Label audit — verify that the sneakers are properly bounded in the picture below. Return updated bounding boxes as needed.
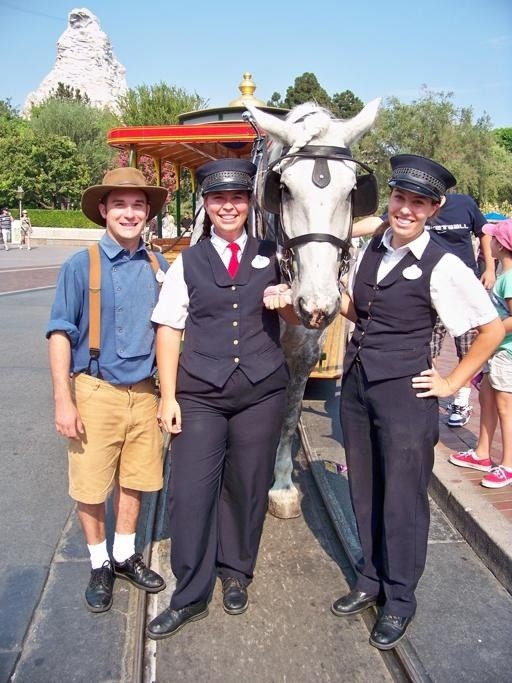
[449,448,492,472]
[447,400,473,428]
[481,462,512,488]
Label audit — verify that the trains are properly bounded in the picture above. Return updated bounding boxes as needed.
[101,66,360,383]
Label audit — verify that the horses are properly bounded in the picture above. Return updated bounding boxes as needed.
[188,95,384,520]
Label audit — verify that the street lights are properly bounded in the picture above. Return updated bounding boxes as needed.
[15,185,26,220]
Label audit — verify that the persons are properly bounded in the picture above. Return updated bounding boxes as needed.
[145,157,305,643]
[45,167,171,617]
[146,207,194,241]
[19,209,35,250]
[351,195,512,488]
[0,209,15,251]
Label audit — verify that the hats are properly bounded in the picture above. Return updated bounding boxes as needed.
[482,218,512,252]
[196,158,257,195]
[79,167,168,226]
[387,153,458,205]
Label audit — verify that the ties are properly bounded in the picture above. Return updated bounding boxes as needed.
[225,238,243,276]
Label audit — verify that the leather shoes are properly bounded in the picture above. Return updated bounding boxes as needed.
[222,574,250,614]
[330,586,374,614]
[368,604,416,650]
[110,552,166,593]
[146,600,209,641]
[83,565,115,613]
